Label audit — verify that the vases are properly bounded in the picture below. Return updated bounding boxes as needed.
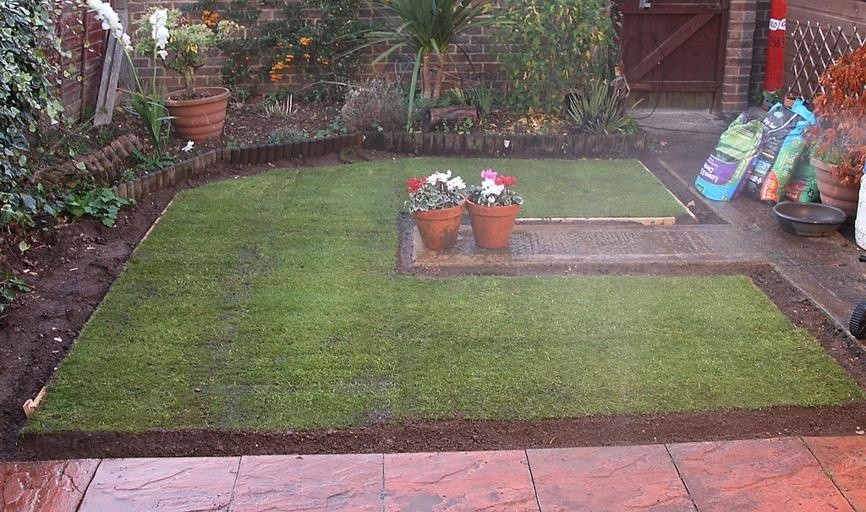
[164,86,232,142]
[809,154,863,225]
[410,198,523,250]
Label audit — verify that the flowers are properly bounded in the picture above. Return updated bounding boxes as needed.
[803,38,866,184]
[405,165,518,214]
[131,4,239,95]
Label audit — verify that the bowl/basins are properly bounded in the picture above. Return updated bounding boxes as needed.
[771,200,847,238]
